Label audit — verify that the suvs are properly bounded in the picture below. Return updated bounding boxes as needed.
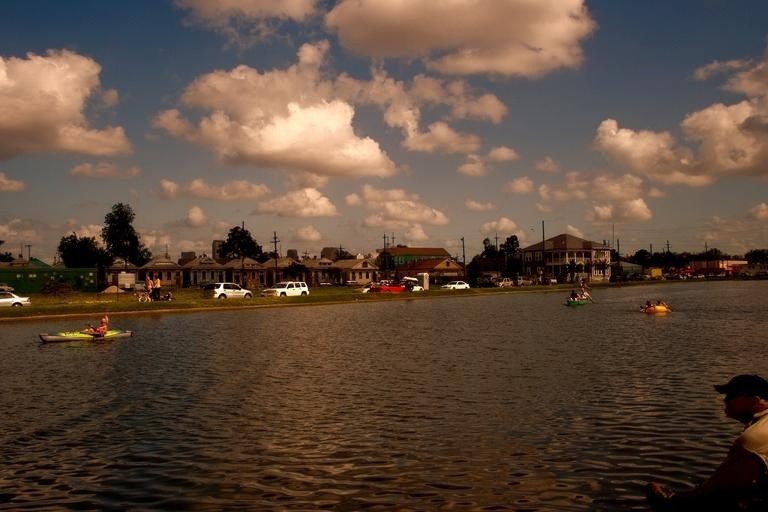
[261,281,310,298]
[203,281,254,300]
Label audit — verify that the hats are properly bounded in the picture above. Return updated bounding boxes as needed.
[711,373,768,399]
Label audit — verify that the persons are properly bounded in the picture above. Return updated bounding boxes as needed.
[80,314,108,337]
[407,280,414,293]
[571,280,592,301]
[145,274,160,301]
[639,296,672,313]
[646,374,768,512]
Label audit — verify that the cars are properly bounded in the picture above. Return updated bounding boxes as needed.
[0,292,33,308]
[441,280,470,291]
[319,282,334,287]
[359,276,425,293]
[663,272,706,280]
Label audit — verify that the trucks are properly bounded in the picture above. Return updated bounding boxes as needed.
[642,268,662,281]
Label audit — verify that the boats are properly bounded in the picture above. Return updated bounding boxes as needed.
[39,328,134,344]
[644,304,672,314]
[566,297,591,306]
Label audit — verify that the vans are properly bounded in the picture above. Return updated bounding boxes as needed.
[344,281,359,285]
[495,277,513,288]
[517,276,534,286]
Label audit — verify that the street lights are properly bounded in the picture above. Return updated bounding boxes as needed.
[461,236,467,283]
